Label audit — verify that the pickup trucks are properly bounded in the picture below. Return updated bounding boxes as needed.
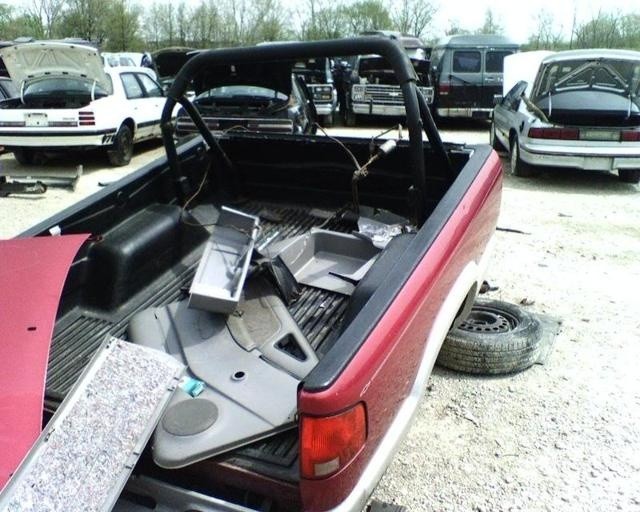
[9,36,505,512]
